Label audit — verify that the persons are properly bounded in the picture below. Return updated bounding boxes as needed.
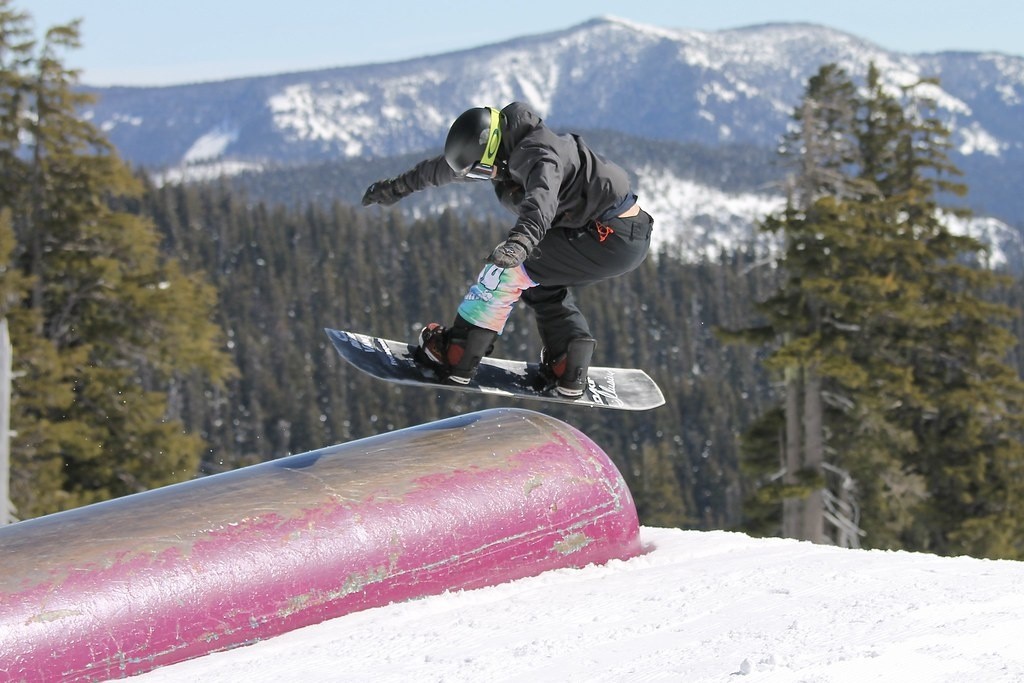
[362,100,655,399]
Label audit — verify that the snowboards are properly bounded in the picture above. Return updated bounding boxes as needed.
[323,325,666,412]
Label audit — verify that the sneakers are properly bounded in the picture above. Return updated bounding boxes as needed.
[537,354,585,398]
[418,322,475,385]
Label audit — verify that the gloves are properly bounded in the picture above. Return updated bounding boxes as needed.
[361,182,381,207]
[485,234,533,268]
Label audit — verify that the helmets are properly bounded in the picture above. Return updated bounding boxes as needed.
[444,108,506,178]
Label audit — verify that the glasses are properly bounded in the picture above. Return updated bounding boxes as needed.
[465,162,493,181]
